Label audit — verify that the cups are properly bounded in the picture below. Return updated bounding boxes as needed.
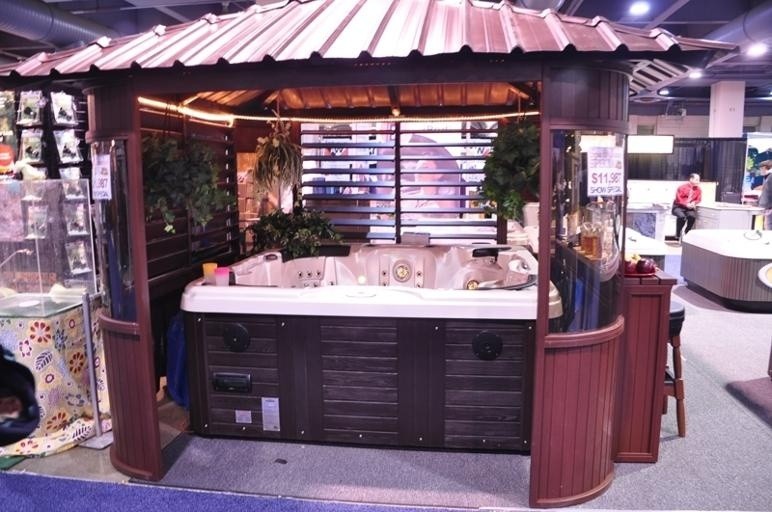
[214,266,230,286]
[202,262,217,284]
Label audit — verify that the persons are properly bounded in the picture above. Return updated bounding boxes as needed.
[758,159,771,231]
[671,172,702,243]
[393,152,450,220]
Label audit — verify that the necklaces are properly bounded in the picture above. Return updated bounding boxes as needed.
[414,194,435,208]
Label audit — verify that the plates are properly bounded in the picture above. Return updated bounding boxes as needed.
[624,272,656,277]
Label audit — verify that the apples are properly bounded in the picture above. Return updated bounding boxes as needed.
[637,260,653,273]
[626,261,638,273]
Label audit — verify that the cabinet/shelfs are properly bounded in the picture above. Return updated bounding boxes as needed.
[695,207,719,230]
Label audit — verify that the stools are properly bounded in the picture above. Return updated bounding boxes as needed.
[665,300,686,436]
